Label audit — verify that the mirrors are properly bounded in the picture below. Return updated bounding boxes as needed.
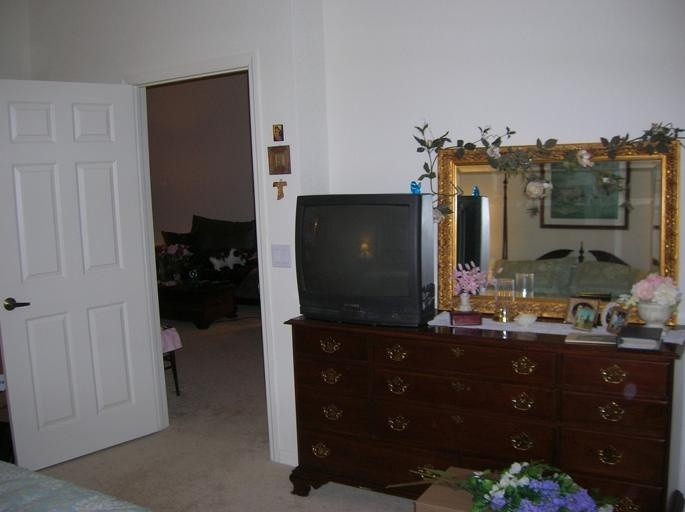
[436,137,681,324]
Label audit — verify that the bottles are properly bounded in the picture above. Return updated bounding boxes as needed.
[471,186,481,196]
[410,181,421,194]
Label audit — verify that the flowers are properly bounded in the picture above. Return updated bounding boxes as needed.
[154,243,194,273]
[383,458,621,512]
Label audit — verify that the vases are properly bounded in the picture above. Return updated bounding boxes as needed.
[158,262,176,283]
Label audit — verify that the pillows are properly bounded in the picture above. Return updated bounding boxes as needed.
[487,259,640,296]
[161,225,253,281]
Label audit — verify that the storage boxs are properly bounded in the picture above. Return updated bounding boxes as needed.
[413,466,501,512]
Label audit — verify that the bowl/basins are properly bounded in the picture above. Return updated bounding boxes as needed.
[514,314,537,327]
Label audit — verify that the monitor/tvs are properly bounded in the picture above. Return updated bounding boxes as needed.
[458,195,488,295]
[294,192,436,328]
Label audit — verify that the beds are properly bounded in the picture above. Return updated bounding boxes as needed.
[485,251,647,303]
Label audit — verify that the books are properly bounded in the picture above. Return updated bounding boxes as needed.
[616,325,663,351]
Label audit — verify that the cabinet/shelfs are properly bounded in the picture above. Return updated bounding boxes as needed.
[282,299,685,512]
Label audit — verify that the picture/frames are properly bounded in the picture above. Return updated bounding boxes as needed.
[538,160,631,231]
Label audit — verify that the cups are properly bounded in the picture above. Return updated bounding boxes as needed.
[516,272,535,299]
[493,279,515,323]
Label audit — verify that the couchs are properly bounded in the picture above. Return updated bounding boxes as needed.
[157,214,260,304]
